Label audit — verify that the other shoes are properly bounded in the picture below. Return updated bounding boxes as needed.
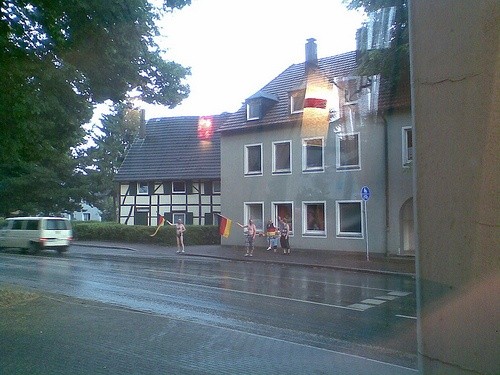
[249,254,252,257]
[244,254,249,257]
[287,249,290,253]
[283,249,286,254]
[181,250,185,253]
[266,247,272,251]
[176,251,181,253]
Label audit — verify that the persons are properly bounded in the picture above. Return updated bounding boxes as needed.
[279,217,291,255]
[166,218,186,253]
[266,219,278,251]
[236,218,256,257]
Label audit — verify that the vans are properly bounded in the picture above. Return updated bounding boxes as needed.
[0,216,73,254]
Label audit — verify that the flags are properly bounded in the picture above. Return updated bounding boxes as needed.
[218,217,232,238]
[150,216,164,236]
[266,227,276,236]
